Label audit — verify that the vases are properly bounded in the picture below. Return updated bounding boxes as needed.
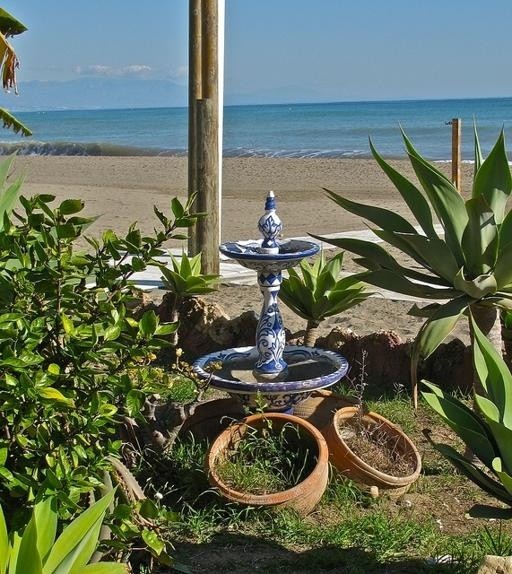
[205,412,330,521]
[293,389,334,419]
[319,406,423,503]
